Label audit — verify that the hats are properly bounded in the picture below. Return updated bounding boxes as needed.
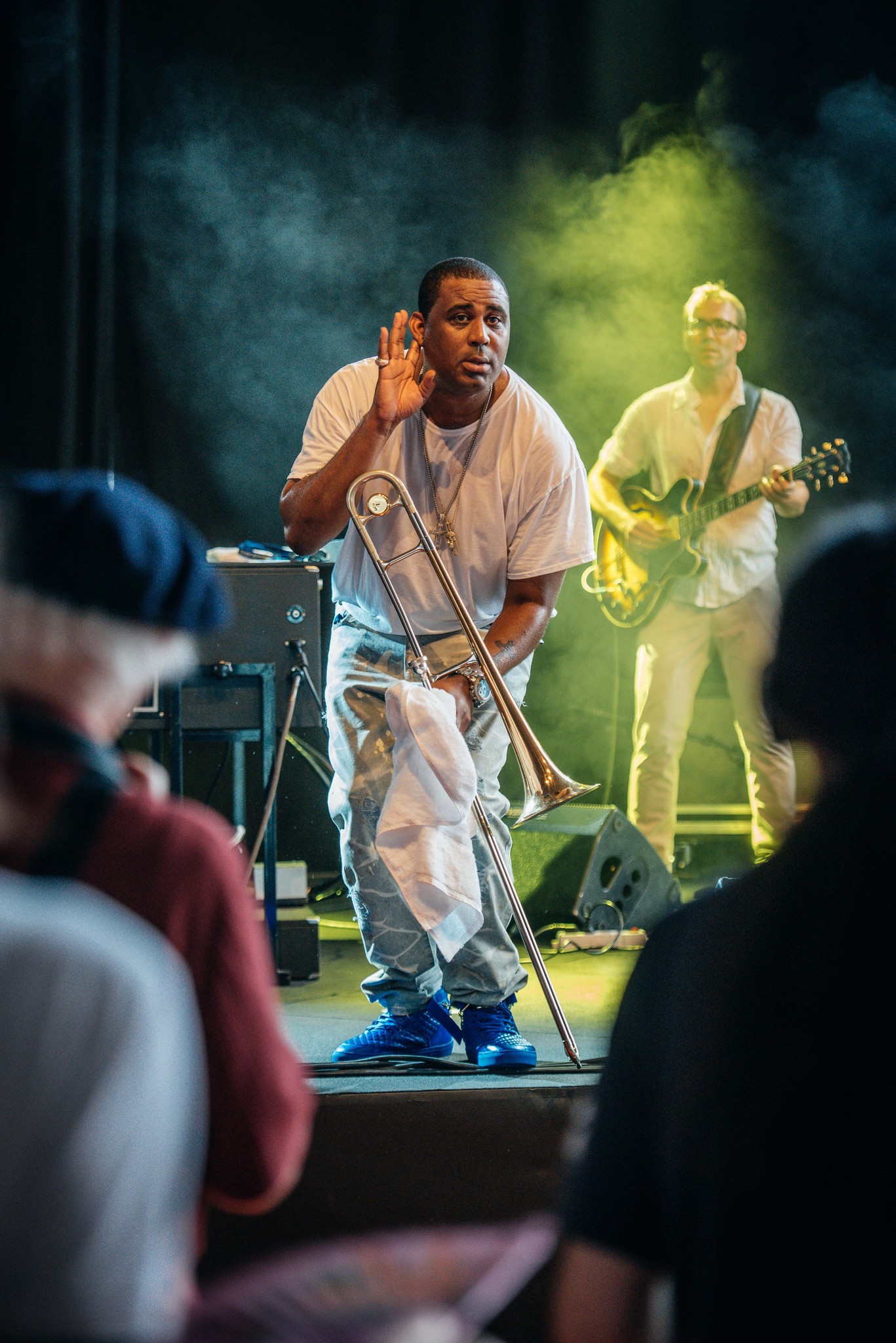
[763,504,896,746]
[0,469,231,638]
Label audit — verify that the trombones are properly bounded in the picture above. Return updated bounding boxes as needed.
[346,469,606,1071]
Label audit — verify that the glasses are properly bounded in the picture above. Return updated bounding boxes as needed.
[684,316,741,335]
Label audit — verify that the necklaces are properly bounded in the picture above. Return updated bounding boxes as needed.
[415,382,493,556]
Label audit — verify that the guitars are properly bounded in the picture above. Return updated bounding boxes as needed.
[590,437,853,628]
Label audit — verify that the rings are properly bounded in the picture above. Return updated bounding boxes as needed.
[374,358,389,366]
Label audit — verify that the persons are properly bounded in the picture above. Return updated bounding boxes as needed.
[279,256,597,1069]
[0,871,208,1343]
[553,517,896,1343]
[0,465,317,1343]
[586,282,809,873]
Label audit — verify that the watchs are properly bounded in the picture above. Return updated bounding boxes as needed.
[446,665,492,708]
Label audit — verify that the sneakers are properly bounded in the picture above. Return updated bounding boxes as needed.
[456,1003,536,1066]
[332,990,462,1064]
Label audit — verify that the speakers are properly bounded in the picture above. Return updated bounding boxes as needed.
[500,800,685,946]
[128,548,321,729]
[193,1074,602,1297]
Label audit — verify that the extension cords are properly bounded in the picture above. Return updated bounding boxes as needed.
[555,927,648,956]
